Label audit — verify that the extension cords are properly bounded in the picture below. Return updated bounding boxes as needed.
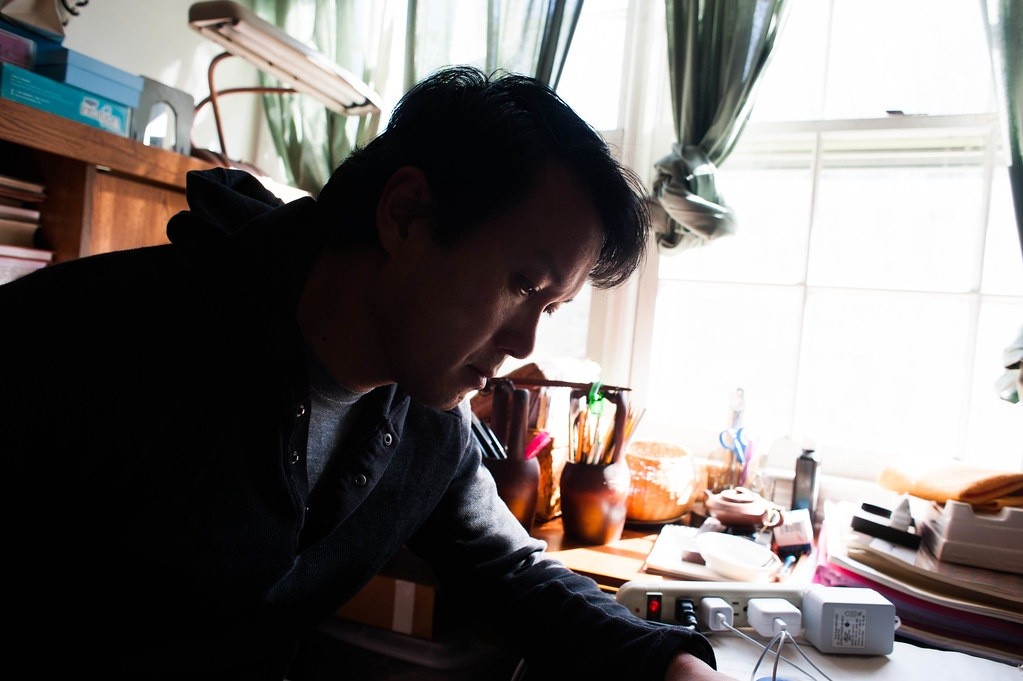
[617,581,824,632]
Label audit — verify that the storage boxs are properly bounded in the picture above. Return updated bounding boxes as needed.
[0,61,128,137]
[45,47,144,109]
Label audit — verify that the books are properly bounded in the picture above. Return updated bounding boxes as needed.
[641,478,1023,672]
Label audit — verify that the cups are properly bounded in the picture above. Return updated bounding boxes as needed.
[559,456,629,549]
[477,453,540,538]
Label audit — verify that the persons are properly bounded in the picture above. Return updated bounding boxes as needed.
[0,62,752,681]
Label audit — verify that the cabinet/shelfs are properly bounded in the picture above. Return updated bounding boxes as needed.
[0,98,229,286]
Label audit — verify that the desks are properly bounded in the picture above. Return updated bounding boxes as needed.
[545,532,1023,681]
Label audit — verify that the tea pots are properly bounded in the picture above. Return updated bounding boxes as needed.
[701,485,784,529]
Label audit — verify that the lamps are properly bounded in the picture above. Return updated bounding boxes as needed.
[188,0,383,182]
